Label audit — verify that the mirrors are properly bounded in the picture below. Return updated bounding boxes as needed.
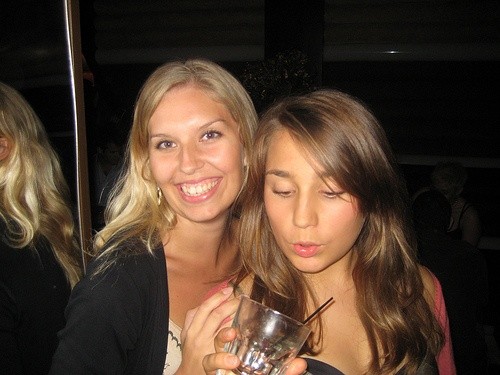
[0,0,93,375]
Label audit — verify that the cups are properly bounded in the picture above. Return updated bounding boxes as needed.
[216,295,311,375]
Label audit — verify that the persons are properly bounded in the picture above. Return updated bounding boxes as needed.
[53,59,259,375]
[206,89,454,374]
[0,82,86,375]
